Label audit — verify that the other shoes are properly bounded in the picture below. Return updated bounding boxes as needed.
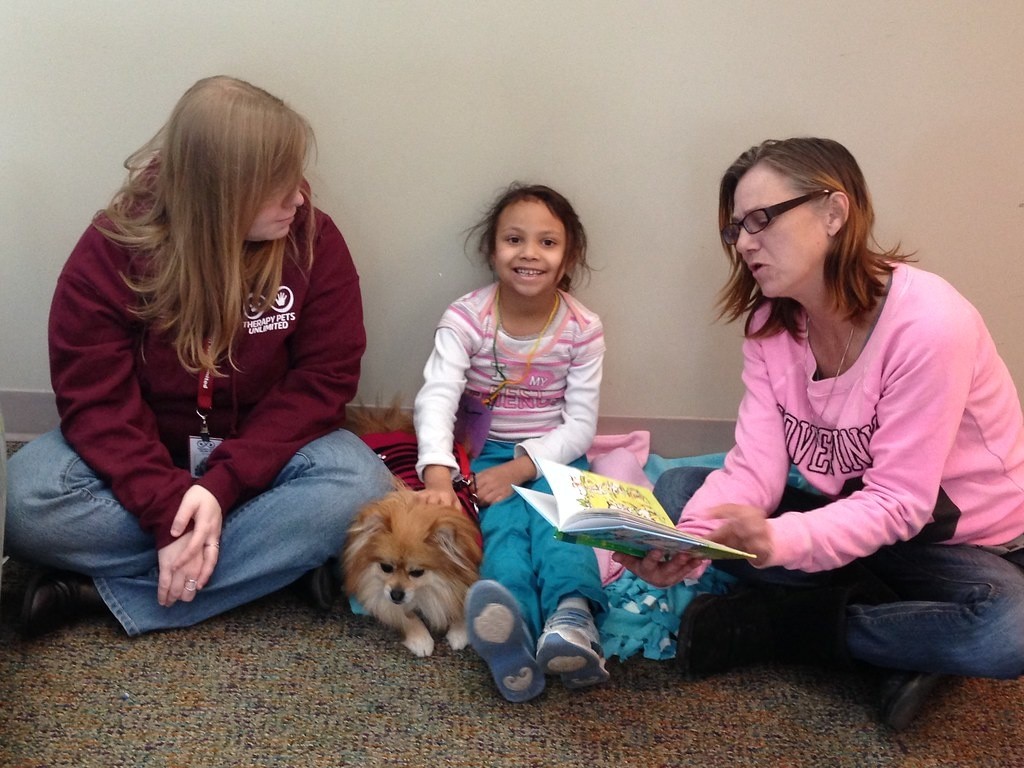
[676,582,836,679]
[812,599,939,731]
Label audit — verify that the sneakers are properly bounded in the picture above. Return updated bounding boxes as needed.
[464,580,545,703]
[536,606,608,691]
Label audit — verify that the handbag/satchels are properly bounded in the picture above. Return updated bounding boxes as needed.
[361,431,483,563]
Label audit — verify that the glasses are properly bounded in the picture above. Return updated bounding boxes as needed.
[719,189,836,245]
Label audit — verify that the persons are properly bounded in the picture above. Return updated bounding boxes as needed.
[415,179,610,703]
[1,75,398,657]
[610,136,1023,729]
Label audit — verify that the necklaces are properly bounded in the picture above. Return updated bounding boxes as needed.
[802,295,856,445]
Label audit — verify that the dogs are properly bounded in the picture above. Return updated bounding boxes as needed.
[342,388,483,658]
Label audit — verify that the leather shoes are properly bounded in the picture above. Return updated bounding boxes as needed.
[284,553,340,613]
[20,563,111,643]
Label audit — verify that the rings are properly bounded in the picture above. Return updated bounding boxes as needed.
[183,579,197,590]
[205,542,220,549]
